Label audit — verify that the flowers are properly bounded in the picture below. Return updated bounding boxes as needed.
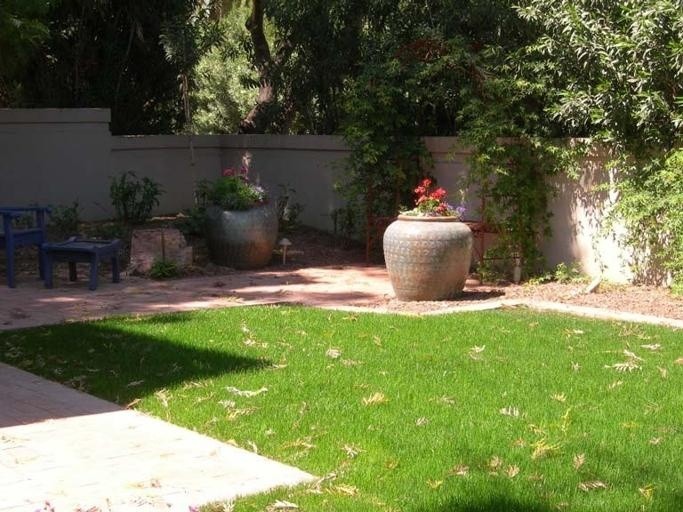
[197,147,272,211]
[397,177,466,219]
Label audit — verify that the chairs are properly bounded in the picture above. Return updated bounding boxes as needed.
[0,205,52,288]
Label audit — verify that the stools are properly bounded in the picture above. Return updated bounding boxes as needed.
[42,235,123,292]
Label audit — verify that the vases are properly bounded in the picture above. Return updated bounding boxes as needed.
[219,206,278,266]
[381,214,475,302]
